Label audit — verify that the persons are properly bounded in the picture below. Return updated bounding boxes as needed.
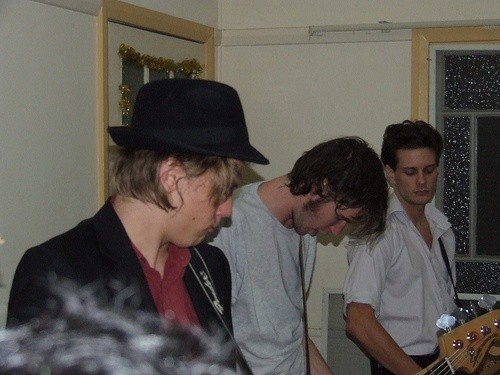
[4,78,254,375]
[204,136,390,375]
[0,278,237,375]
[343,119,457,375]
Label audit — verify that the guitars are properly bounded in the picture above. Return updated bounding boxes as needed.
[415,293,500,375]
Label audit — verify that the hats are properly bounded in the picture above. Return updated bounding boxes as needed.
[107,77,270,164]
